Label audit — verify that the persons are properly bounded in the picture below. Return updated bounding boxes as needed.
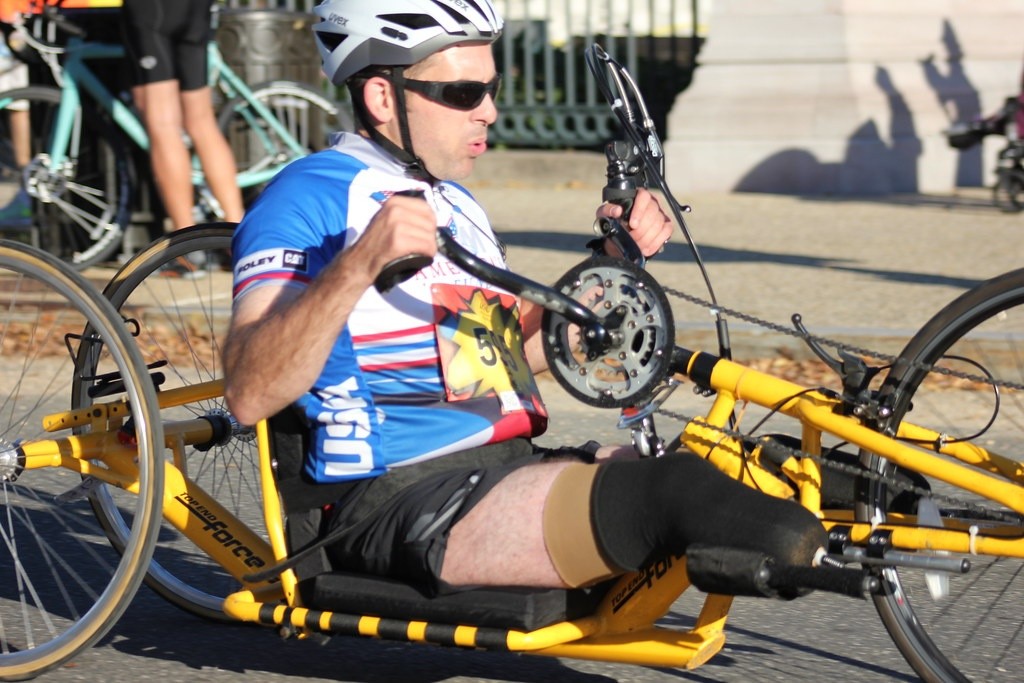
[0,0,246,280]
[221,0,934,600]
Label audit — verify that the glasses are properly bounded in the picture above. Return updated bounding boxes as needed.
[358,68,503,111]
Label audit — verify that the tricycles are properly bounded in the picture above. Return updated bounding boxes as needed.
[0,39,1024,683]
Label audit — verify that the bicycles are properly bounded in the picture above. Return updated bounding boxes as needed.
[2,9,358,278]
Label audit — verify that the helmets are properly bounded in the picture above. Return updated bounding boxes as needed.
[309,0,504,87]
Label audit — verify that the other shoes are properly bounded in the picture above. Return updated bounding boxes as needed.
[116,247,206,280]
